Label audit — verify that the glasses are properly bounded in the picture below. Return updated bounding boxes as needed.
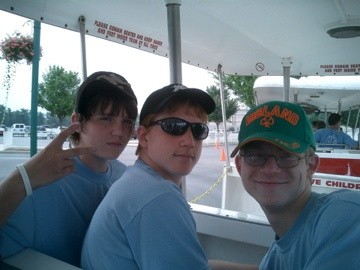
[240,150,309,170]
[150,116,209,142]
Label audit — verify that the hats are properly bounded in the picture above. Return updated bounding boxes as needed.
[138,83,216,119]
[229,100,315,153]
[74,71,135,101]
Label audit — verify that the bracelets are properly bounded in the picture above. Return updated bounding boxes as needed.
[16,163,32,196]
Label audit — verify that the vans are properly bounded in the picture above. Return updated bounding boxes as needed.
[29,126,47,140]
[11,123,25,137]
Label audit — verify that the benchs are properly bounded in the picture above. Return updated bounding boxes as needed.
[315,143,360,177]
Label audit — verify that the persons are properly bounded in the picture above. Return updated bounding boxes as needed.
[310,113,360,149]
[80,82,259,270]
[229,100,360,270]
[0,70,139,270]
